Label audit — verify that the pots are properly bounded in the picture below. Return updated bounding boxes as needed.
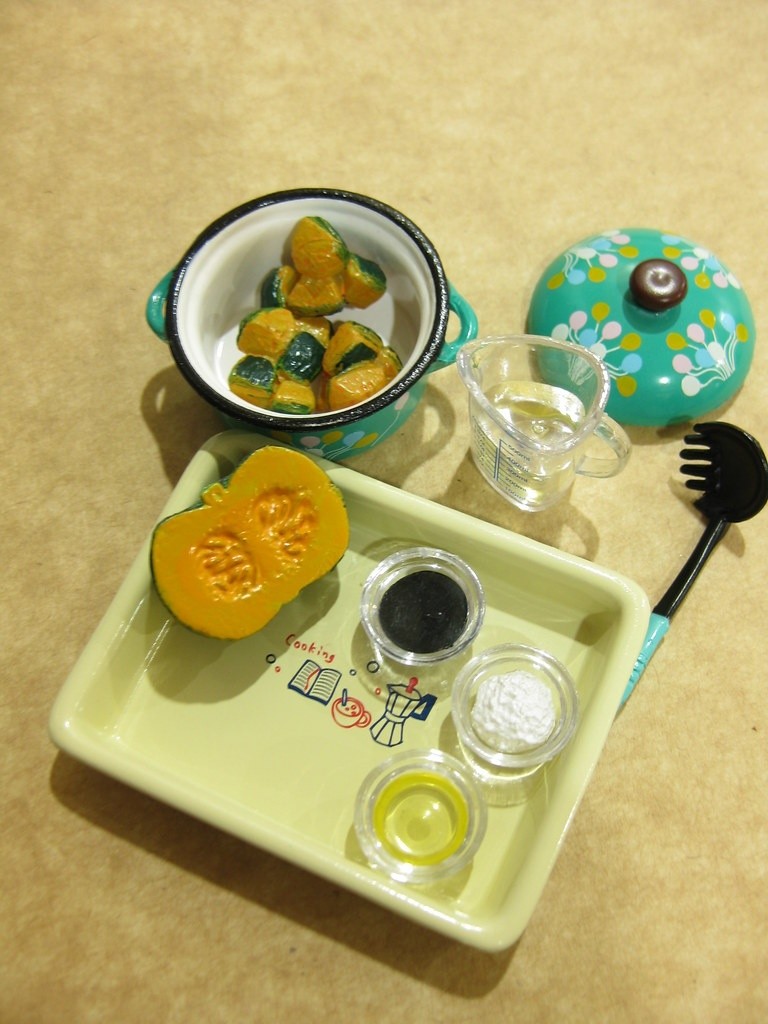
[144,188,479,462]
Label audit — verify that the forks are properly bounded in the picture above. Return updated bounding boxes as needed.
[621,421,768,704]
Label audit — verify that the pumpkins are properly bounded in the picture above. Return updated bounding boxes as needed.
[227,218,408,417]
[148,444,350,641]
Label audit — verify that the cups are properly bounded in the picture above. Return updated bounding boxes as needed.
[456,335,632,513]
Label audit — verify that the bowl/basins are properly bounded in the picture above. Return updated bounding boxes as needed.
[359,546,485,665]
[354,749,487,886]
[449,642,580,768]
